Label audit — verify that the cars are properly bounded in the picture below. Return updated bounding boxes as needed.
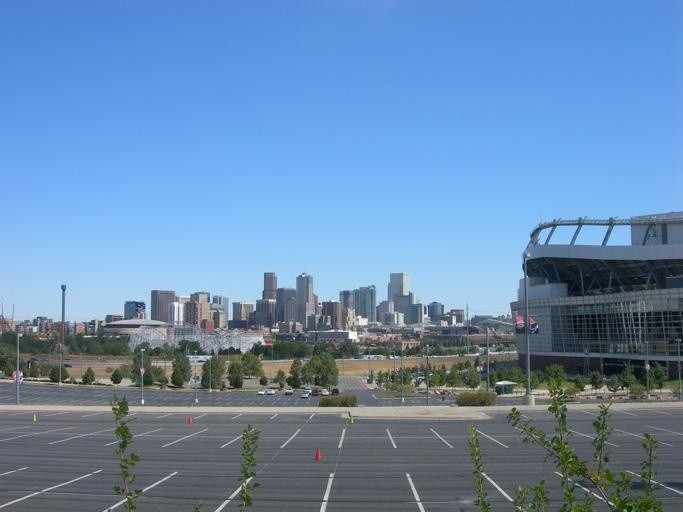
[257,385,340,398]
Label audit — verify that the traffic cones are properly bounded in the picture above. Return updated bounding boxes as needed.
[188,416,191,424]
[316,448,320,461]
